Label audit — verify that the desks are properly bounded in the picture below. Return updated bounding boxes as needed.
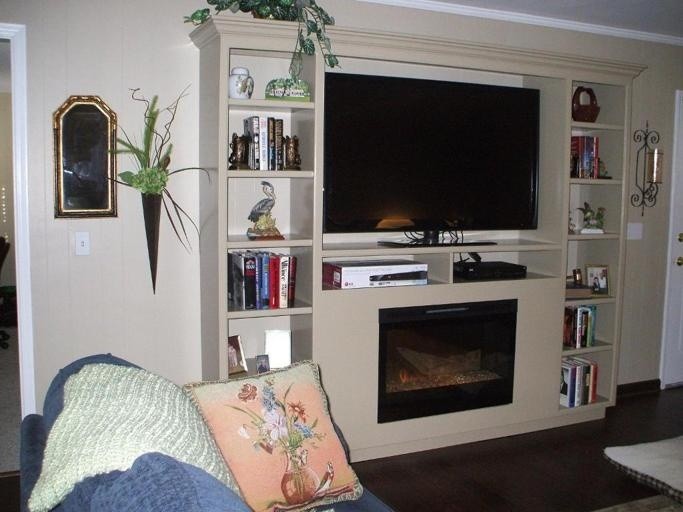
[602,438,683,500]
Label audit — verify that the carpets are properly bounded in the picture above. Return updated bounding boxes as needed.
[592,494,683,512]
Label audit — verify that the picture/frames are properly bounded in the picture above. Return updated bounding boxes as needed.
[256,354,270,374]
[584,263,611,298]
[229,335,249,375]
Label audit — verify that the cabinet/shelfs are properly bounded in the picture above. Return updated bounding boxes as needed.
[200,47,648,462]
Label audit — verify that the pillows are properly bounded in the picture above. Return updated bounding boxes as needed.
[182,360,365,511]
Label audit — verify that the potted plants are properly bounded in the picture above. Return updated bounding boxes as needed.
[183,0,342,99]
[108,88,211,295]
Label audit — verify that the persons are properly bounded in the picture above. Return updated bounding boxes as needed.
[599,272,607,288]
[592,276,600,294]
[560,369,568,396]
[257,359,268,373]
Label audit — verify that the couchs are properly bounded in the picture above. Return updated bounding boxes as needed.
[18,356,394,512]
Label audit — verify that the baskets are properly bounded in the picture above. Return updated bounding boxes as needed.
[572,87,601,122]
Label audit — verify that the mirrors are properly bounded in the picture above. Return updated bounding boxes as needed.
[51,93,118,217]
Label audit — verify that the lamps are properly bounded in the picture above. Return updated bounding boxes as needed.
[631,121,664,216]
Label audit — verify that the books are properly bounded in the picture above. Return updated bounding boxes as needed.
[570,135,599,180]
[558,304,598,408]
[227,250,298,311]
[243,116,284,172]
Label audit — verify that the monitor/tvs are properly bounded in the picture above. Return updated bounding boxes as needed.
[324,72,541,249]
